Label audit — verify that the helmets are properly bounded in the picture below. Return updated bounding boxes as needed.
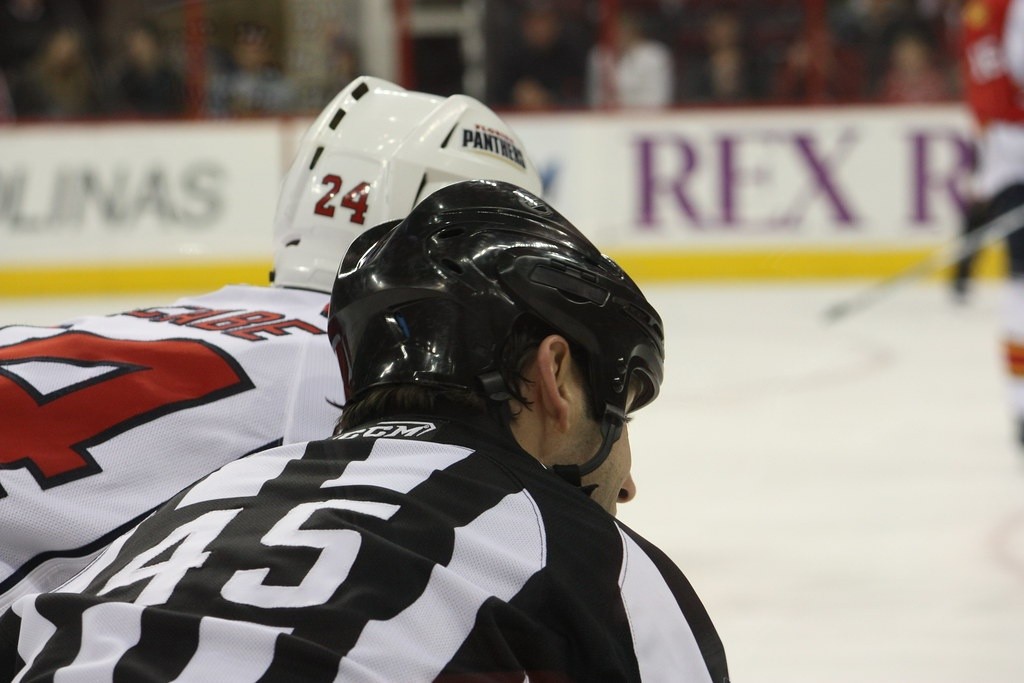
[327,179,665,424]
[271,76,543,297]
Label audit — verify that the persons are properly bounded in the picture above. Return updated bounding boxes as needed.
[0,74,542,613]
[0,21,358,120]
[0,179,730,683]
[945,0,1024,444]
[484,0,958,111]
